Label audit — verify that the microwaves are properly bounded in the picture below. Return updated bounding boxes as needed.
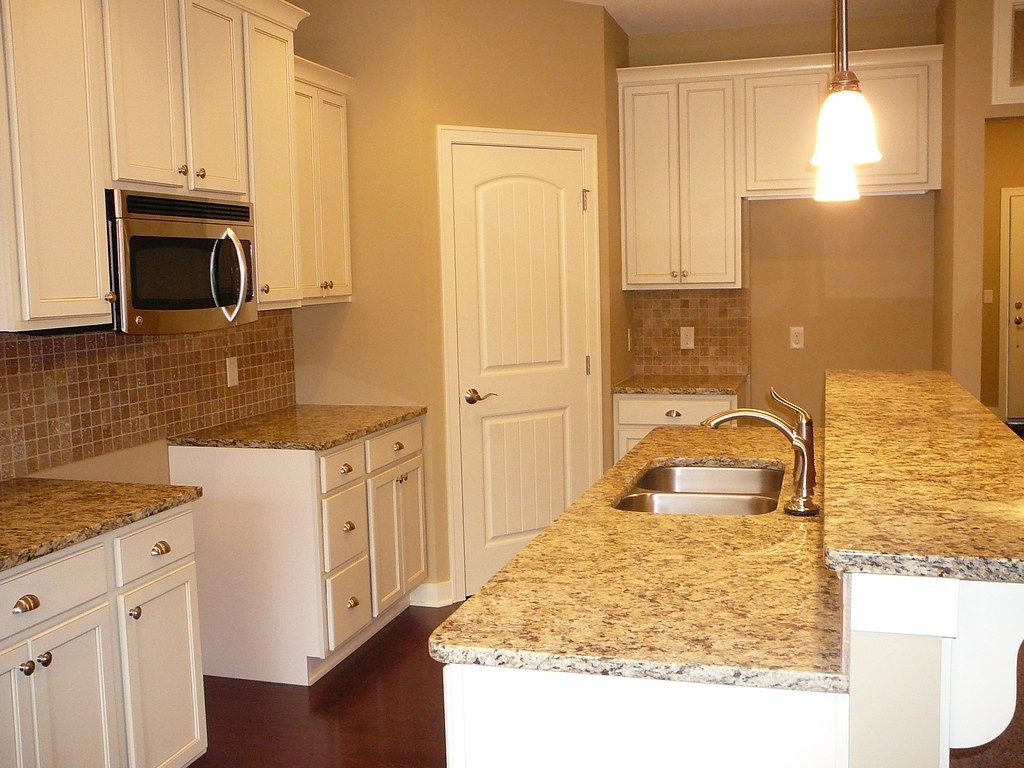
[111,188,259,336]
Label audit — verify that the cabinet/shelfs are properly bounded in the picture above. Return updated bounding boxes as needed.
[616,44,943,290]
[168,416,428,686]
[0,0,353,334]
[612,380,747,466]
[0,501,208,768]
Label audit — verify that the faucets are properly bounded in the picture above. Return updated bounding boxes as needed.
[699,384,823,518]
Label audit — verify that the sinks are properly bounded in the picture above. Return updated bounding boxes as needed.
[609,493,780,517]
[632,455,788,493]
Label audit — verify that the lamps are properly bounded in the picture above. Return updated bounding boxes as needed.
[813,0,859,202]
[810,0,884,166]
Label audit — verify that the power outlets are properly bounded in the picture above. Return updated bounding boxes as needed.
[790,326,804,349]
[680,327,694,349]
